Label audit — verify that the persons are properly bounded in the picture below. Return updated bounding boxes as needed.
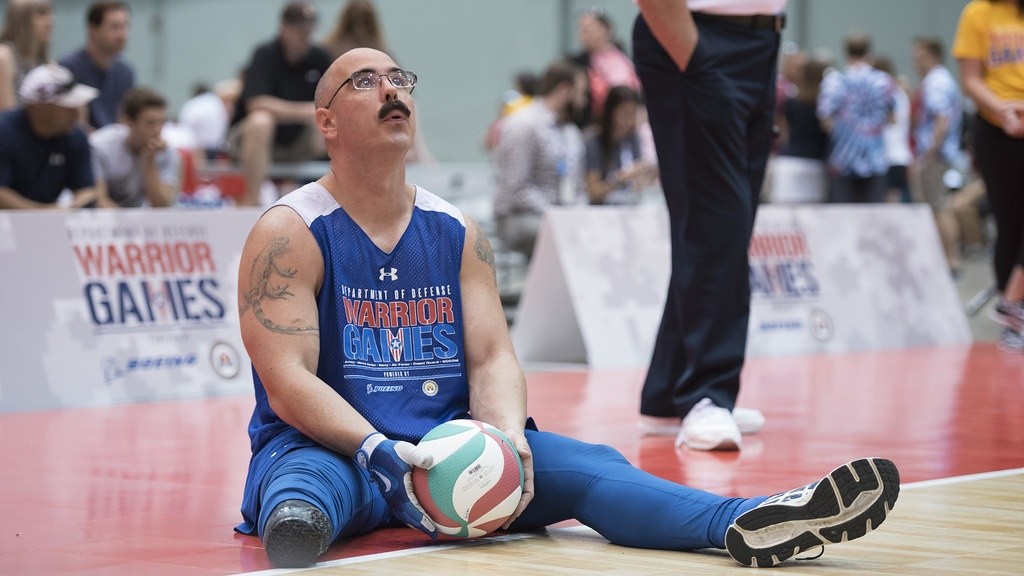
[0,0,990,277]
[949,0,1024,341]
[232,46,899,568]
[631,1,788,450]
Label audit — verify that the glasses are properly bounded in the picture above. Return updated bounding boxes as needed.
[324,70,417,109]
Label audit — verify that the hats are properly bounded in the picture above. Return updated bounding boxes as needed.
[283,3,316,22]
[21,63,98,108]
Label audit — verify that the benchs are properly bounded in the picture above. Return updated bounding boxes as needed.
[189,157,332,203]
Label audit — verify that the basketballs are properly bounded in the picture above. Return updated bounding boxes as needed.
[412,418,526,540]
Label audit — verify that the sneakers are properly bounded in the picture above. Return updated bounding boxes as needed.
[266,500,330,567]
[986,295,1024,336]
[638,405,765,434]
[676,399,740,451]
[726,458,901,567]
[997,326,1024,354]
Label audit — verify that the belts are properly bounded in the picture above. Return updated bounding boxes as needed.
[694,11,786,31]
